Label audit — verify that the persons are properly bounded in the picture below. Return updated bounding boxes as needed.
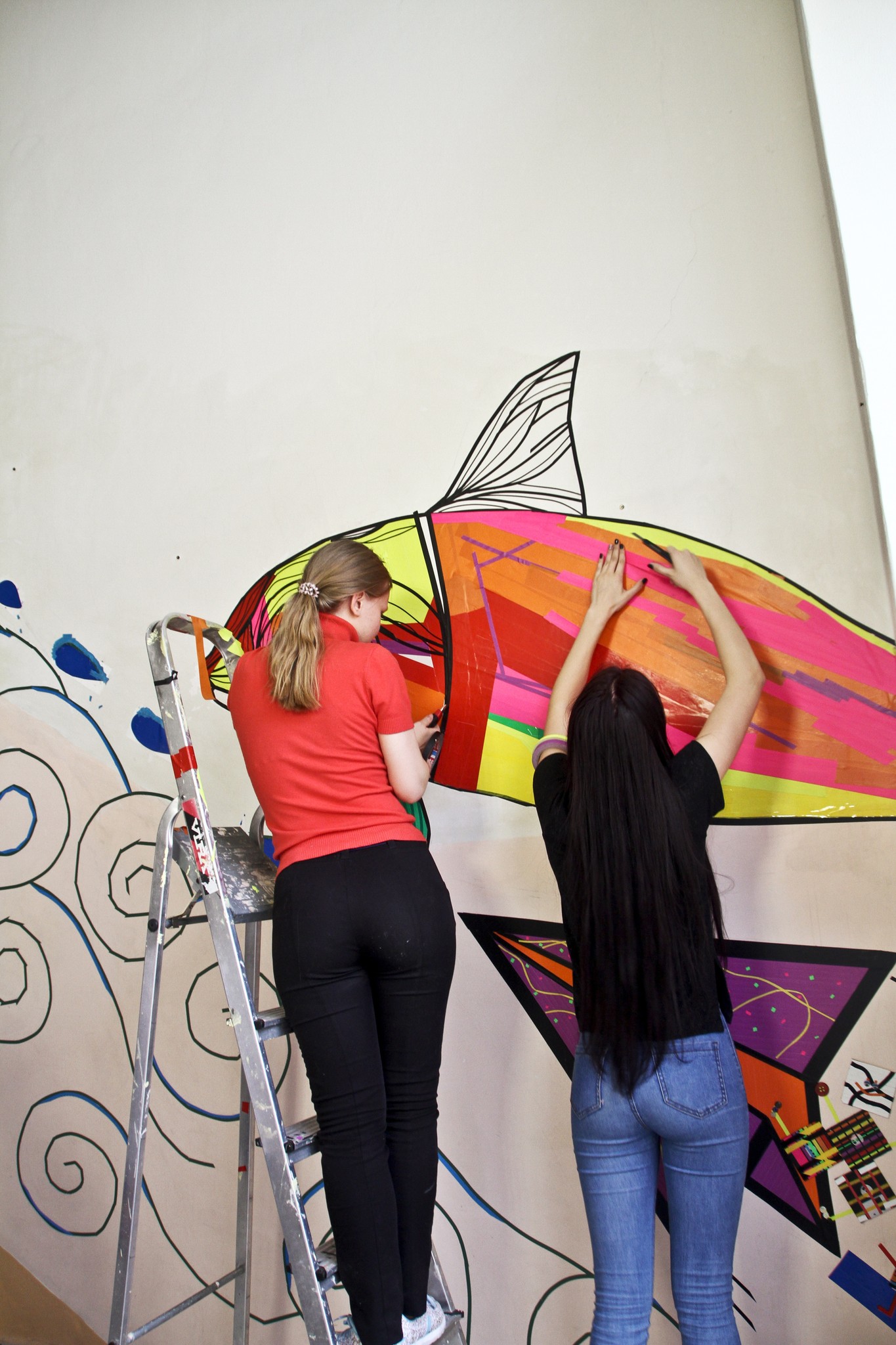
[228,542,449,1344]
[531,536,767,1345]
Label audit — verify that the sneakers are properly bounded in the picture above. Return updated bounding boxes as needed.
[400,1293,445,1345]
[335,1326,409,1345]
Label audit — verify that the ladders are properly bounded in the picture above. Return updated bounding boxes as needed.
[106,616,466,1345]
